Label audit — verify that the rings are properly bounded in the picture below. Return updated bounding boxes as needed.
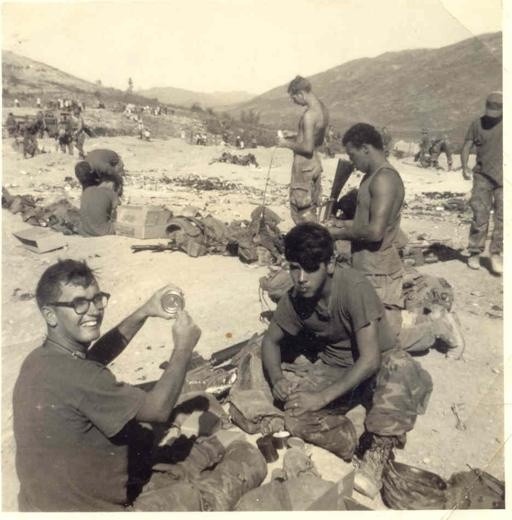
[293,403,299,409]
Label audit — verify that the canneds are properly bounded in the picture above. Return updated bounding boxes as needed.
[286,435,305,451]
[159,290,186,316]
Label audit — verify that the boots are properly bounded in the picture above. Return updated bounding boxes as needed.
[354,436,392,499]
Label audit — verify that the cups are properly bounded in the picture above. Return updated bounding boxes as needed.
[256,430,290,464]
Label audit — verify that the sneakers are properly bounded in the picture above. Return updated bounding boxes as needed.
[491,254,503,273]
[284,449,319,478]
[467,253,480,268]
[430,306,464,359]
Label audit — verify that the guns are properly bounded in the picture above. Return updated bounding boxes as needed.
[132,331,268,391]
[130,245,177,252]
[321,159,355,225]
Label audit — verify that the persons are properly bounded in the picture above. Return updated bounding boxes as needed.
[273,74,329,222]
[412,126,453,172]
[316,123,348,160]
[10,258,267,511]
[461,89,503,278]
[192,133,207,146]
[379,126,394,158]
[5,95,169,238]
[259,223,435,499]
[220,131,260,150]
[324,121,464,361]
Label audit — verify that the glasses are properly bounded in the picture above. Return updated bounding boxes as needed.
[48,292,110,314]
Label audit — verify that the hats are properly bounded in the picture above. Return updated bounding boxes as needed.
[485,93,502,118]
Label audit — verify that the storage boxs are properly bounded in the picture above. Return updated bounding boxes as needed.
[111,203,171,239]
